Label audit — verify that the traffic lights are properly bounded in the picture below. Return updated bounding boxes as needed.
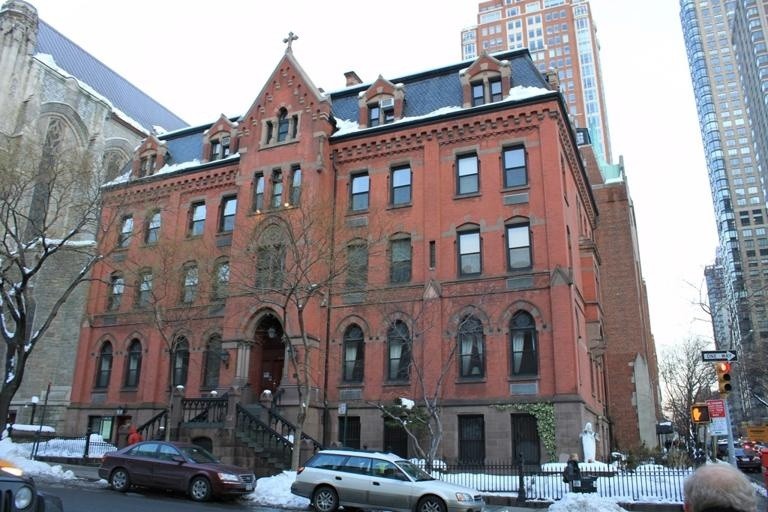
[690,404,710,424]
[715,361,732,398]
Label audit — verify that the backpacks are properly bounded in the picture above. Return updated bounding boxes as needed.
[563,467,571,483]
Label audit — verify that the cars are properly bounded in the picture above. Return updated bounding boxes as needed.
[98,441,257,502]
[718,439,768,473]
[290,449,486,512]
[0,467,63,512]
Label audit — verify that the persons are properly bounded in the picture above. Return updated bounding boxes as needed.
[682,464,758,512]
[128,424,144,445]
[567,453,583,494]
